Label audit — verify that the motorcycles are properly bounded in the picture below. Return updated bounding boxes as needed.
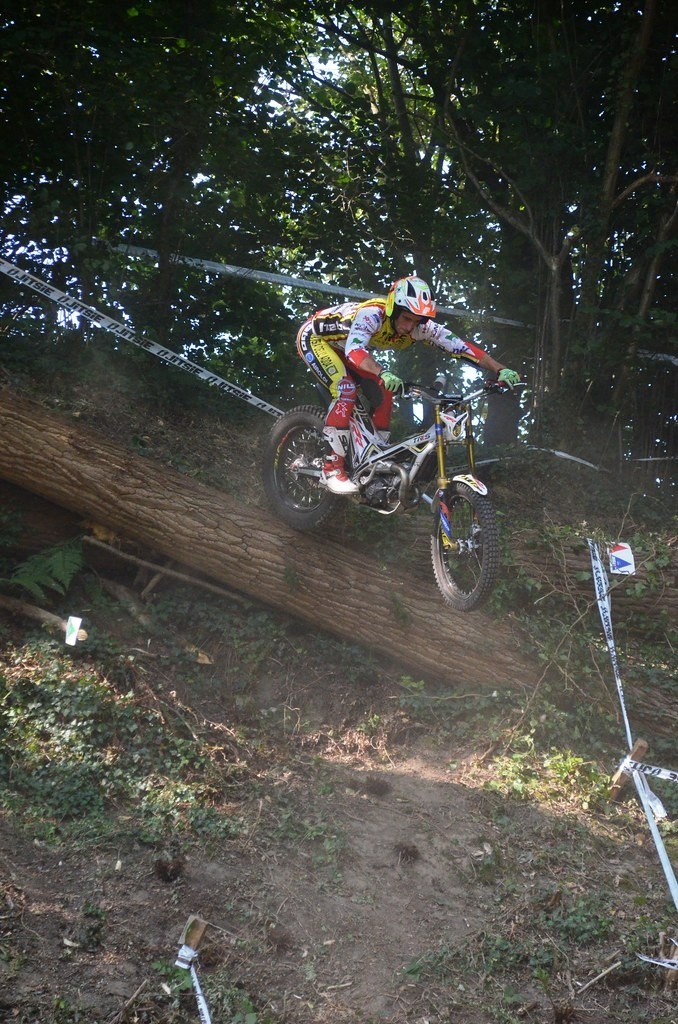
[262,367,530,613]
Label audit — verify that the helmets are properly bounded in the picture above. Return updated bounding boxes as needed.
[385,276,437,324]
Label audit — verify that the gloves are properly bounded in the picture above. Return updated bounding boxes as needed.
[378,368,405,399]
[496,367,521,391]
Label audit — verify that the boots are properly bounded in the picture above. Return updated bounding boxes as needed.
[378,430,391,444]
[318,426,360,495]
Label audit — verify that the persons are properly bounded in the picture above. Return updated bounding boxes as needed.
[296,277,521,494]
